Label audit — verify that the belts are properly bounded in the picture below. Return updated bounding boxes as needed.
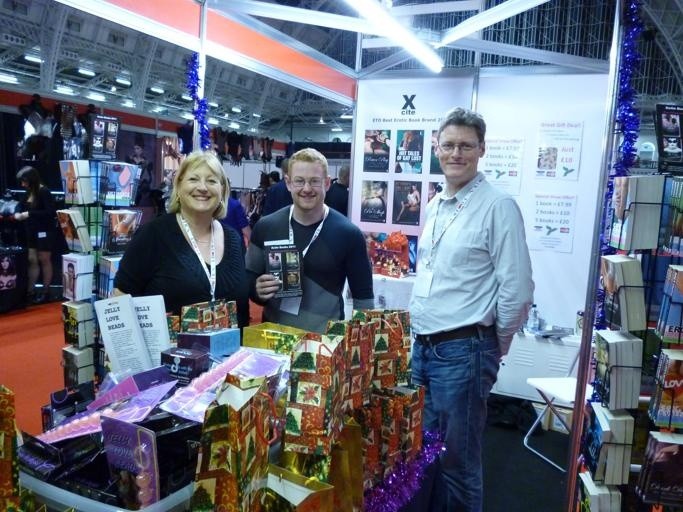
[416,324,496,345]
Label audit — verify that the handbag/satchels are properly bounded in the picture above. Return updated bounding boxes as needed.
[167,299,238,343]
[189,376,268,512]
[1,385,19,512]
[244,322,305,354]
[268,308,424,512]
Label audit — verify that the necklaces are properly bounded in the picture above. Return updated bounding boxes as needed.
[184,223,217,244]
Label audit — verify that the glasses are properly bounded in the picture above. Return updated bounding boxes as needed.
[292,175,326,187]
[437,141,480,151]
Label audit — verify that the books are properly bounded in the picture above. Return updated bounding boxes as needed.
[135,295,170,368]
[655,102,683,176]
[91,293,153,383]
[263,240,302,299]
[86,112,122,162]
[575,171,683,511]
[57,158,143,388]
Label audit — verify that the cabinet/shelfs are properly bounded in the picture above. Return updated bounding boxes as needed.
[567,110,683,512]
[61,148,140,398]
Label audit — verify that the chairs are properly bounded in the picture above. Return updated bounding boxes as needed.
[525,337,585,475]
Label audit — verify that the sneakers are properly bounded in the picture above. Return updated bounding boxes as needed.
[27,293,51,301]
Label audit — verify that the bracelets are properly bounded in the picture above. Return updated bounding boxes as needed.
[383,138,389,143]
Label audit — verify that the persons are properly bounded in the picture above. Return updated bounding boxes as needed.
[395,184,420,222]
[18,93,54,160]
[409,106,534,508]
[128,134,148,165]
[107,141,115,150]
[363,130,389,154]
[0,257,17,289]
[242,146,376,337]
[663,137,682,154]
[218,153,351,243]
[21,165,56,267]
[93,137,103,149]
[362,181,387,208]
[111,151,252,336]
